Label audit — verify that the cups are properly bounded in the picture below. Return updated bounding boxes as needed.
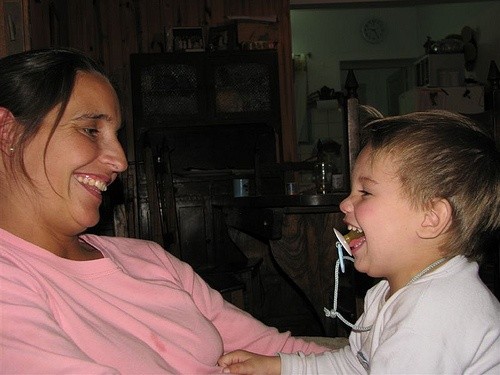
[283,171,300,207]
[332,175,343,190]
[313,162,332,194]
[232,179,250,197]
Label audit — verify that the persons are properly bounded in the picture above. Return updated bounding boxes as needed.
[217,109,500,375]
[0,48,331,375]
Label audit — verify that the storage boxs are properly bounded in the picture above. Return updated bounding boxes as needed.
[229,19,279,48]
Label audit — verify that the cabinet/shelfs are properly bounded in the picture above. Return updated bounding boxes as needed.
[124,49,283,318]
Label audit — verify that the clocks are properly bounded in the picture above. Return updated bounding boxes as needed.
[361,16,386,43]
